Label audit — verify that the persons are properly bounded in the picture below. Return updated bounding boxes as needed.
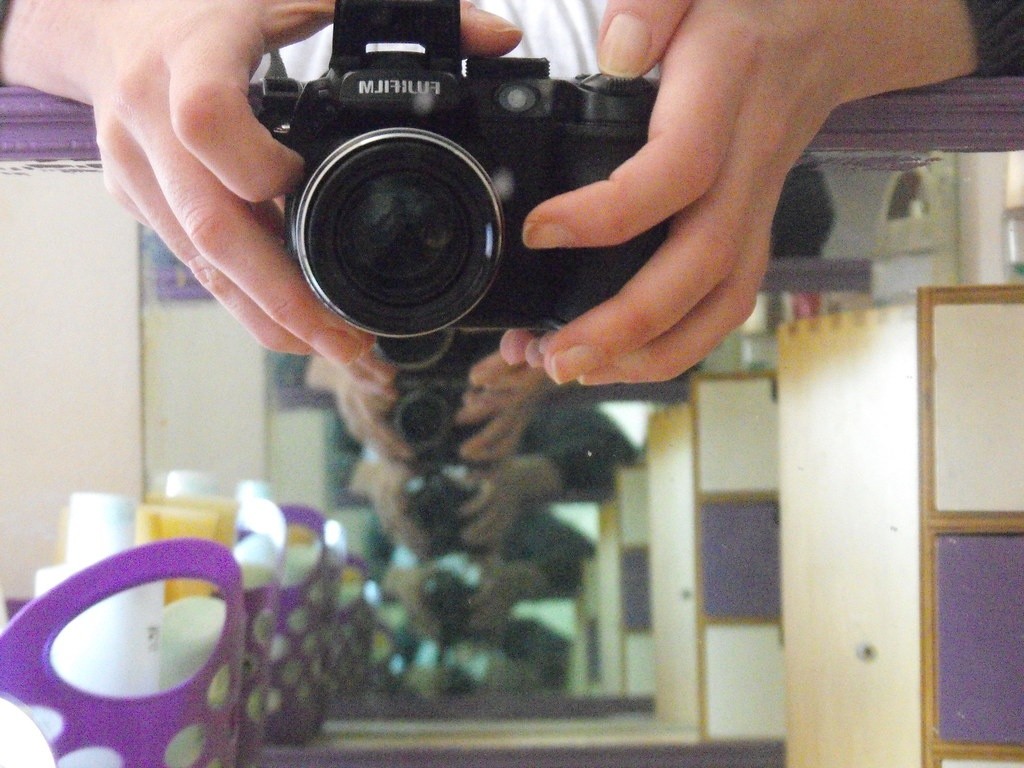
[265,351,639,697]
[1,0,1024,391]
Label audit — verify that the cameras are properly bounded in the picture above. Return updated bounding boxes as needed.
[252,0,669,341]
[370,337,489,710]
